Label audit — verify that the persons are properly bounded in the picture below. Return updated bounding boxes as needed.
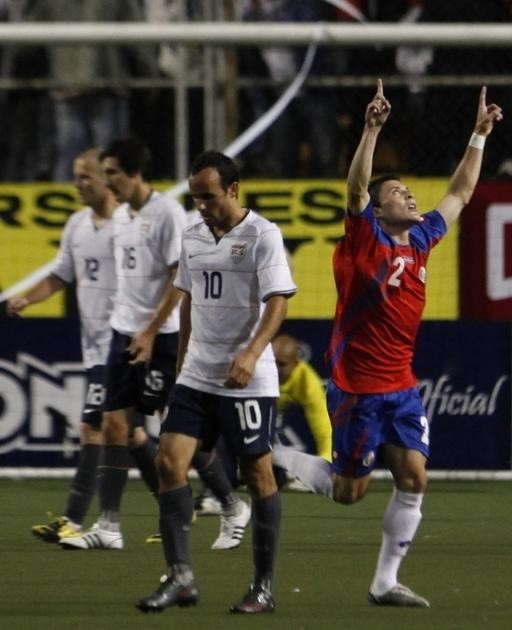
[2,0,158,179]
[269,335,334,492]
[137,150,298,615]
[269,77,503,608]
[58,142,251,551]
[0,147,198,544]
[236,0,468,173]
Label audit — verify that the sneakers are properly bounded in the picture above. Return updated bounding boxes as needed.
[209,501,252,552]
[196,492,226,515]
[57,522,125,552]
[28,514,84,542]
[365,581,432,610]
[136,572,201,613]
[146,510,198,544]
[228,580,279,615]
